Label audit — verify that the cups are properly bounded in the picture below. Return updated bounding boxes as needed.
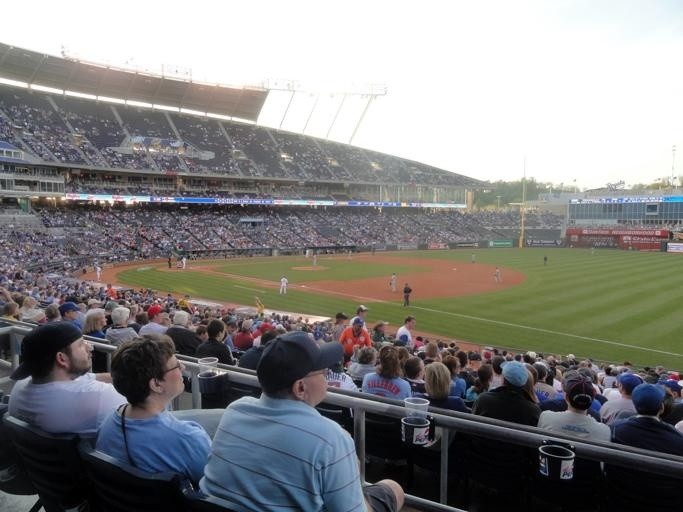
[403,396,429,424]
[197,356,220,378]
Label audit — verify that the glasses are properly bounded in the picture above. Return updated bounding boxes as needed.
[160,361,180,373]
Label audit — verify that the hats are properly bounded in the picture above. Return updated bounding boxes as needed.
[658,379,683,391]
[242,319,254,330]
[148,304,162,316]
[256,331,344,390]
[357,305,369,314]
[88,299,101,306]
[632,384,666,410]
[58,302,81,312]
[616,374,641,389]
[350,316,364,326]
[10,322,83,380]
[336,312,348,319]
[500,361,528,387]
[563,375,597,405]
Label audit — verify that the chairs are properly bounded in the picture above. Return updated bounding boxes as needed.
[0,327,682,511]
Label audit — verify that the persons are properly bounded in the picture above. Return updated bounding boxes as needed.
[0,102,682,511]
